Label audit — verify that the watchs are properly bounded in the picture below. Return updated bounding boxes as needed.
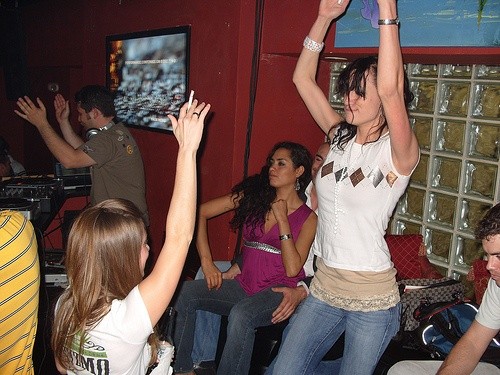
[376,16,400,26]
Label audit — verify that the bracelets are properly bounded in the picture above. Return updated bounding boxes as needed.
[279,234,293,242]
[302,35,325,54]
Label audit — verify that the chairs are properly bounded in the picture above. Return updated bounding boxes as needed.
[382,231,448,288]
[213,313,286,375]
[401,259,492,333]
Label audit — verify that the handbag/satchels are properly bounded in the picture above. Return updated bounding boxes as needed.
[392,277,466,337]
[385,230,426,281]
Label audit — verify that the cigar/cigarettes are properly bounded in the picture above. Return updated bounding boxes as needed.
[186,90,196,114]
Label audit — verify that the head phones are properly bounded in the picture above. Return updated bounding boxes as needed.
[86,116,120,140]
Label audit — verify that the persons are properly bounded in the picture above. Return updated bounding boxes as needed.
[0,139,26,178]
[160,140,318,375]
[186,140,332,375]
[274,0,422,375]
[0,207,43,375]
[13,83,150,230]
[385,203,500,375]
[49,99,212,375]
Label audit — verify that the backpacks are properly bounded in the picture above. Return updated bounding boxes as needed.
[422,300,500,366]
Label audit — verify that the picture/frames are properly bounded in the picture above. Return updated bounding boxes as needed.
[104,24,193,134]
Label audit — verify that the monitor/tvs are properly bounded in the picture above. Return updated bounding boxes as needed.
[105,24,192,135]
